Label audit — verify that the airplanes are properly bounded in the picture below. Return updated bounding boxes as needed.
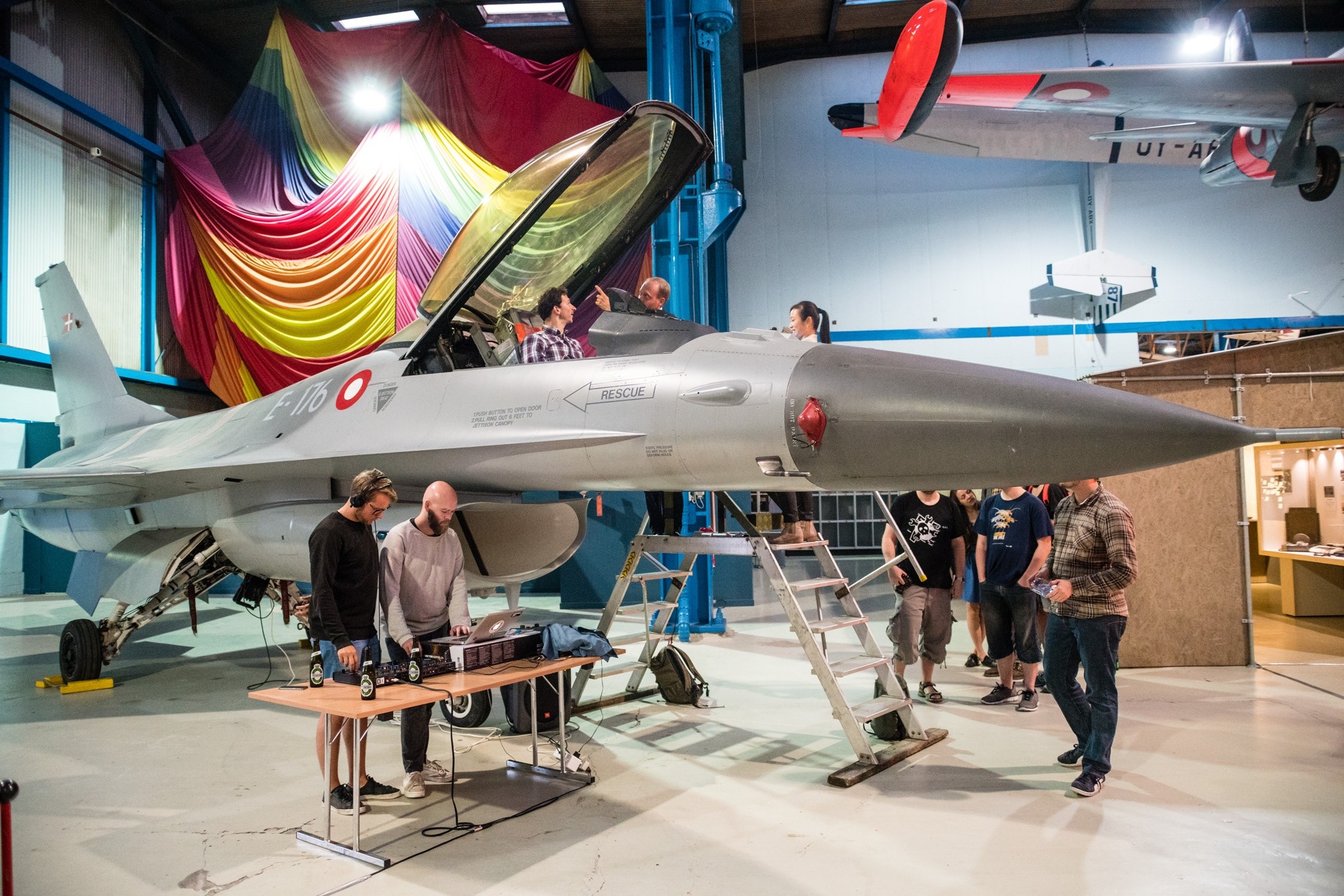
[820,0,1344,205]
[2,98,1343,735]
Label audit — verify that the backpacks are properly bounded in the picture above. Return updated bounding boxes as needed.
[863,675,909,741]
[649,636,710,705]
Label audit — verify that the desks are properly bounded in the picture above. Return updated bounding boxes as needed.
[245,646,629,868]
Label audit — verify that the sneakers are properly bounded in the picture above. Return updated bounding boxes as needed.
[1070,772,1105,797]
[982,656,998,668]
[1017,688,1040,711]
[964,654,979,668]
[800,520,817,542]
[1012,660,1024,680]
[766,521,804,544]
[321,785,366,815]
[1034,671,1049,687]
[403,771,425,798]
[421,759,457,782]
[1057,743,1084,767]
[347,775,401,801]
[984,668,999,677]
[1041,685,1051,694]
[981,681,1018,704]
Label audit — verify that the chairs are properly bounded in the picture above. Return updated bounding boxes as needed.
[508,305,545,341]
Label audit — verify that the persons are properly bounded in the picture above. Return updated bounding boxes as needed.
[1022,483,1069,693]
[949,489,997,668]
[972,486,1055,711]
[770,301,832,544]
[296,469,402,816]
[520,284,585,363]
[595,277,683,537]
[882,491,966,702]
[984,620,1023,679]
[1027,478,1138,797]
[380,481,471,798]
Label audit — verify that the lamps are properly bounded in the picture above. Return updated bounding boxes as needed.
[1187,1,1214,54]
[1328,446,1333,450]
[1294,449,1300,453]
[1164,335,1177,354]
[1310,448,1316,452]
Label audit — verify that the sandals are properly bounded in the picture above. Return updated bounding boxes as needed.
[918,681,942,702]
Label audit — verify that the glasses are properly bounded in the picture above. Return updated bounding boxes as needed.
[366,502,391,515]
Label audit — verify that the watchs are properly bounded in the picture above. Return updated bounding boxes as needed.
[955,577,965,583]
